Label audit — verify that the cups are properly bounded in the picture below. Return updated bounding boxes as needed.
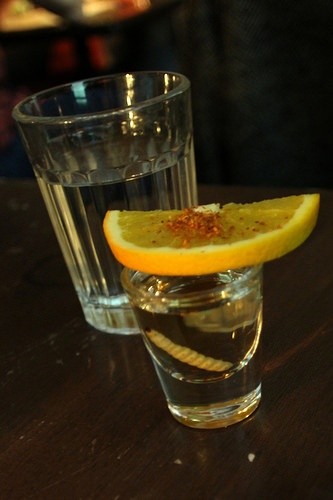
[119,265,268,430]
[13,68,202,337]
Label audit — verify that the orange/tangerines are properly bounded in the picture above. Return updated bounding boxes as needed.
[103,194,319,277]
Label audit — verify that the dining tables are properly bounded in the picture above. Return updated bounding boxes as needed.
[1,176,333,500]
